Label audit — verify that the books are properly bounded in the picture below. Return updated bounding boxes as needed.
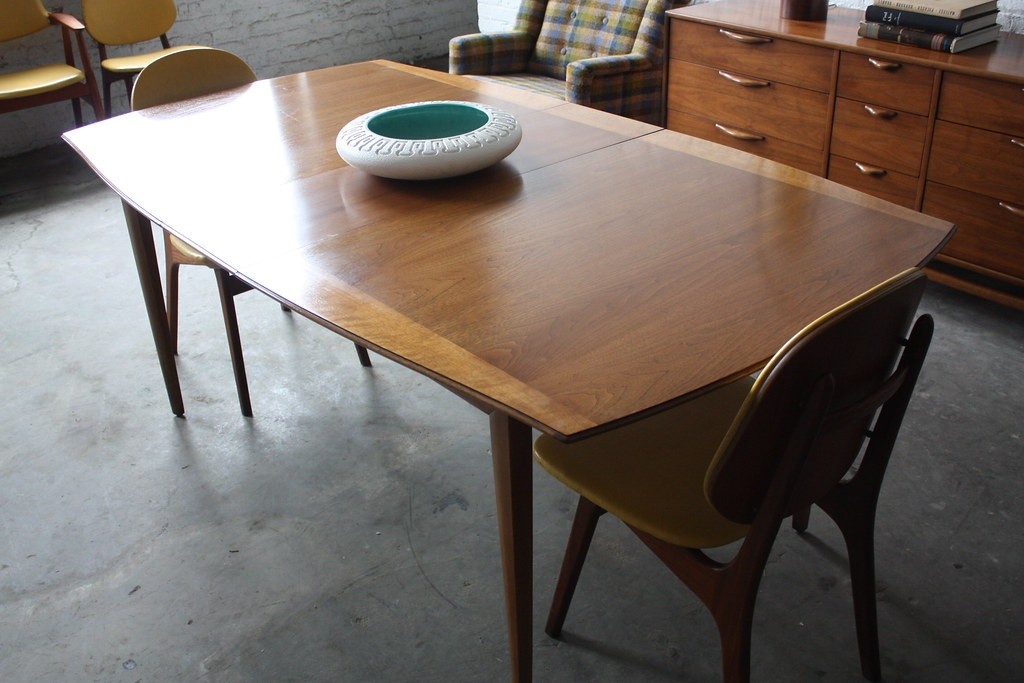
[857,0,1001,52]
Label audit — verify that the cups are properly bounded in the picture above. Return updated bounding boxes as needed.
[778,0,829,21]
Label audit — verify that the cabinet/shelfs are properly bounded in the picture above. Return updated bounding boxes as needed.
[665,2,1024,313]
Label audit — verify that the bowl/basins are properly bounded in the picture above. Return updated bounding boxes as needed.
[335,99,523,181]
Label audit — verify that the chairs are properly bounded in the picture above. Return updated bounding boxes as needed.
[0,0,104,127]
[82,0,212,120]
[532,266,935,683]
[130,49,373,416]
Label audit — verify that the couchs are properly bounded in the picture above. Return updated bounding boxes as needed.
[447,1,687,127]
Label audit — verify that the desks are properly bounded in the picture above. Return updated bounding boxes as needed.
[62,59,956,683]
